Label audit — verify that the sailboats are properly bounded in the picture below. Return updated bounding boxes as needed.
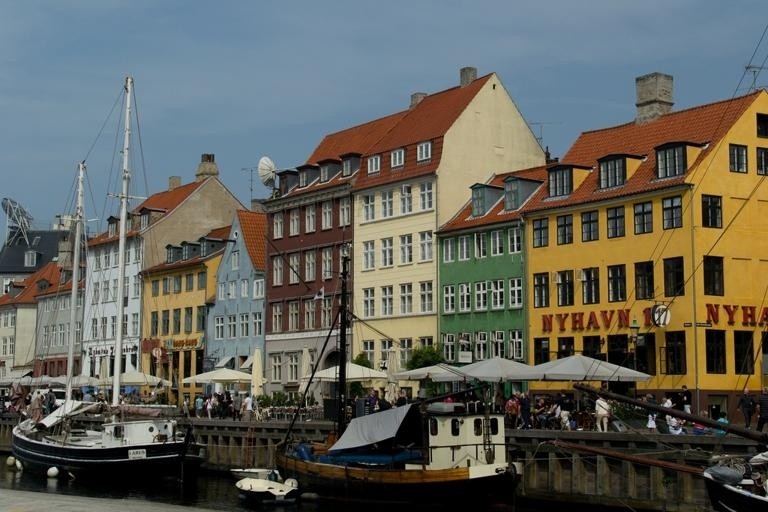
[9,75,208,506]
[526,23,768,510]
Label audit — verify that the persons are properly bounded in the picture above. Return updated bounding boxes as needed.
[448,385,768,432]
[46,388,56,414]
[25,390,34,405]
[191,389,254,423]
[32,391,46,417]
[368,389,379,413]
[73,386,155,404]
[395,390,407,407]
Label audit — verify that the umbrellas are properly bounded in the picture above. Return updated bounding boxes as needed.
[181,366,269,395]
[299,359,388,401]
[11,374,101,390]
[391,361,466,385]
[534,351,652,411]
[97,367,173,398]
[456,355,545,404]
